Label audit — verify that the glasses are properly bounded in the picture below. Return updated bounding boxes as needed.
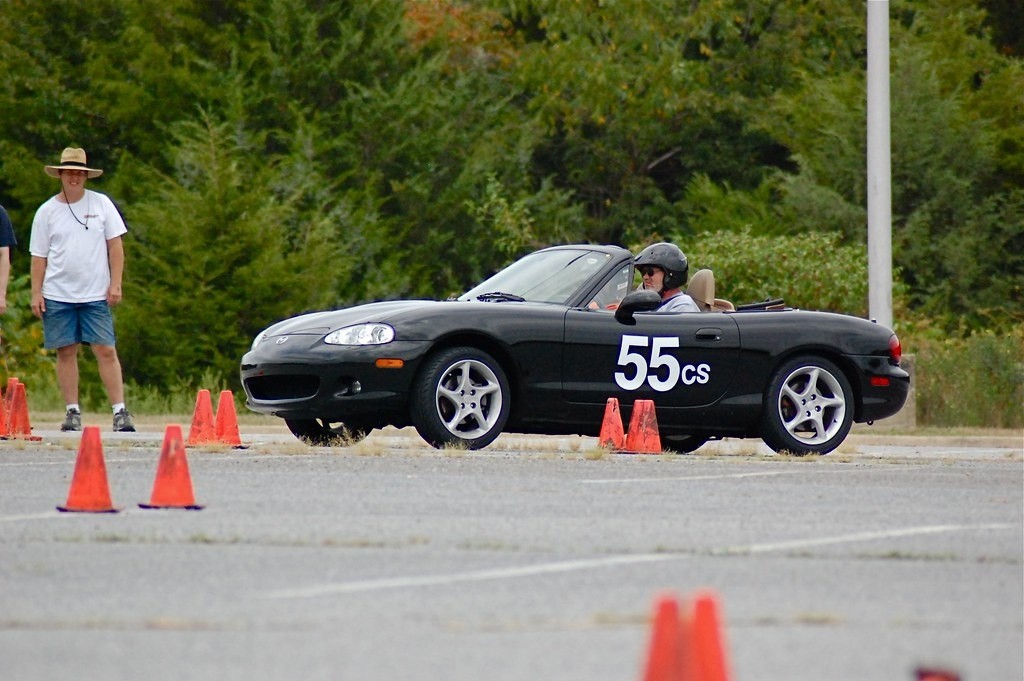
[641,268,663,276]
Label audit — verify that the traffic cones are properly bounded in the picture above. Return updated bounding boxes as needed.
[0,377,43,441]
[214,389,250,450]
[137,422,206,510]
[617,399,665,455]
[182,390,215,448]
[56,425,119,513]
[641,593,733,681]
[594,397,625,455]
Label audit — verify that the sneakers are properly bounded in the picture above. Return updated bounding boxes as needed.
[61,408,81,431]
[113,408,136,432]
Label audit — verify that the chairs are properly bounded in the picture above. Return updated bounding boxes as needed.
[685,269,715,311]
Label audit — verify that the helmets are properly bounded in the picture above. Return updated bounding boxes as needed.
[634,243,688,289]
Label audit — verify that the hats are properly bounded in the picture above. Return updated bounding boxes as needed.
[45,147,103,178]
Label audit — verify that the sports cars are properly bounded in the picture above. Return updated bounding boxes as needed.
[240,244,911,459]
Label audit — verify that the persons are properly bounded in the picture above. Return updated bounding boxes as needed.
[0,206,17,313]
[633,242,701,312]
[28,147,135,432]
